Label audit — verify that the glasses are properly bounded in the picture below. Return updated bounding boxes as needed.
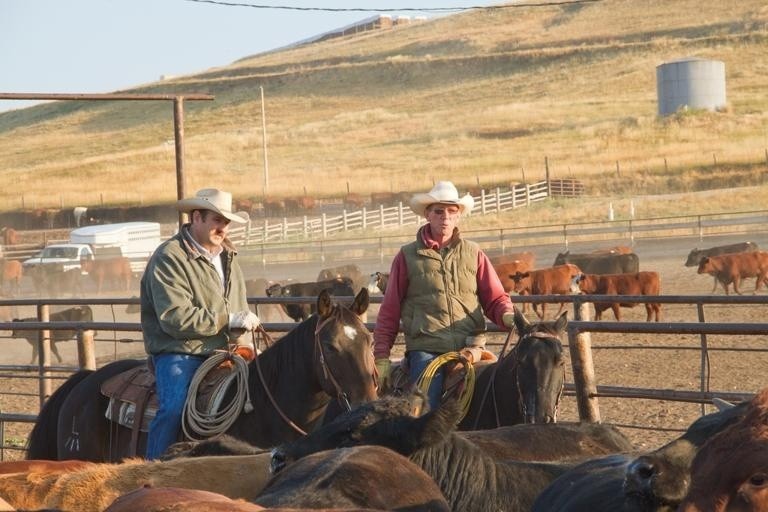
[433,208,458,214]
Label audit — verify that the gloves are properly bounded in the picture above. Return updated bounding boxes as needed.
[375,359,393,391]
[228,310,260,332]
[502,313,529,335]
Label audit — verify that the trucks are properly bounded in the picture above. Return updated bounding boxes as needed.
[23,221,161,276]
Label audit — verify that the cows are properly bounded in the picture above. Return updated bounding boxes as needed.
[684,242,759,293]
[697,249,768,296]
[489,247,666,322]
[0,257,133,293]
[126,297,141,314]
[24,206,157,228]
[244,263,391,322]
[3,226,17,244]
[11,303,97,364]
[1,385,768,512]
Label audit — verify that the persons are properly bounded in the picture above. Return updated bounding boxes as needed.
[375,180,529,411]
[140,188,261,459]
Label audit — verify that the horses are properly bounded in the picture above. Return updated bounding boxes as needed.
[19,286,378,462]
[384,305,568,425]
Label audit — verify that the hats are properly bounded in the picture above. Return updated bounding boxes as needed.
[410,180,474,216]
[176,188,250,224]
